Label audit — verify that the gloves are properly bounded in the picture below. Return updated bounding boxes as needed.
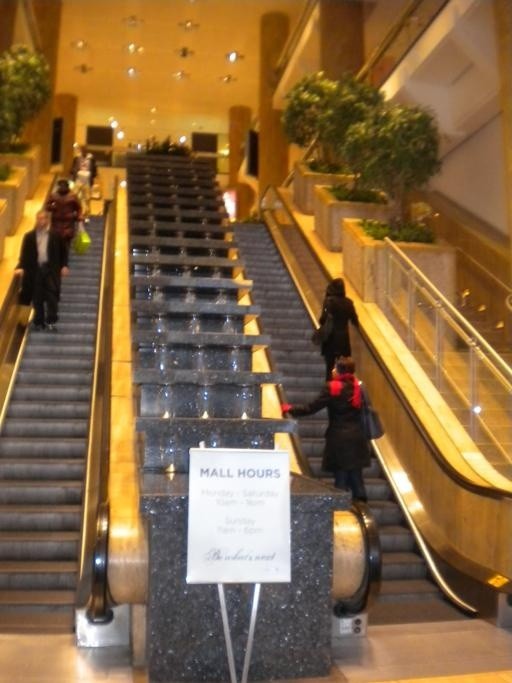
[281,401,292,413]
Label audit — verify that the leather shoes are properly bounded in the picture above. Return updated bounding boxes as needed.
[35,324,45,331]
[46,323,58,332]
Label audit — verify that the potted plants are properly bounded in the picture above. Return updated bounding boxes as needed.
[281,70,456,303]
[0,43,52,261]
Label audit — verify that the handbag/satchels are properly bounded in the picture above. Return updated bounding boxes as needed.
[73,221,91,254]
[312,313,335,345]
[358,378,384,439]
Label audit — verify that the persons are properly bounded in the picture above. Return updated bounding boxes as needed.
[319,277,359,382]
[280,354,371,507]
[75,157,92,222]
[71,141,98,213]
[144,133,189,156]
[46,177,83,265]
[16,208,71,330]
